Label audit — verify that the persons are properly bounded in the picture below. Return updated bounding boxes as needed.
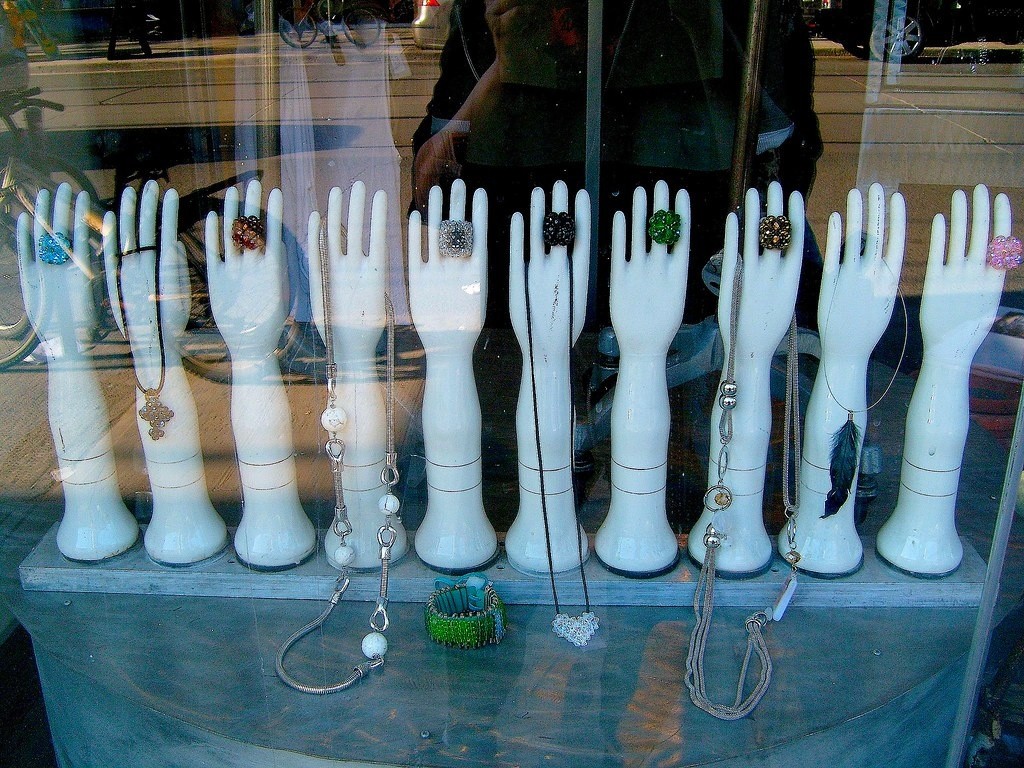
[292,0,339,42]
[409,1,825,516]
[0,5,30,93]
[18,179,1013,578]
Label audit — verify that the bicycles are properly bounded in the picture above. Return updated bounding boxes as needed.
[278,0,381,49]
[0,85,314,385]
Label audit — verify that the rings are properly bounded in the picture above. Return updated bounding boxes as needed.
[544,211,577,245]
[986,235,1023,270]
[759,216,791,250]
[439,219,472,259]
[231,215,265,250]
[39,233,72,265]
[648,210,681,244]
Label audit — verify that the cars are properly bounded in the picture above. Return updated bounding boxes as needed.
[817,0,1024,65]
[410,0,452,49]
[799,7,826,39]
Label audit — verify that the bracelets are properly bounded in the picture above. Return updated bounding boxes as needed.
[424,572,507,649]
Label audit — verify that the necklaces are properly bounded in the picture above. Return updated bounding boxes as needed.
[116,245,175,439]
[820,258,908,519]
[524,254,599,647]
[274,217,400,693]
[683,250,801,720]
